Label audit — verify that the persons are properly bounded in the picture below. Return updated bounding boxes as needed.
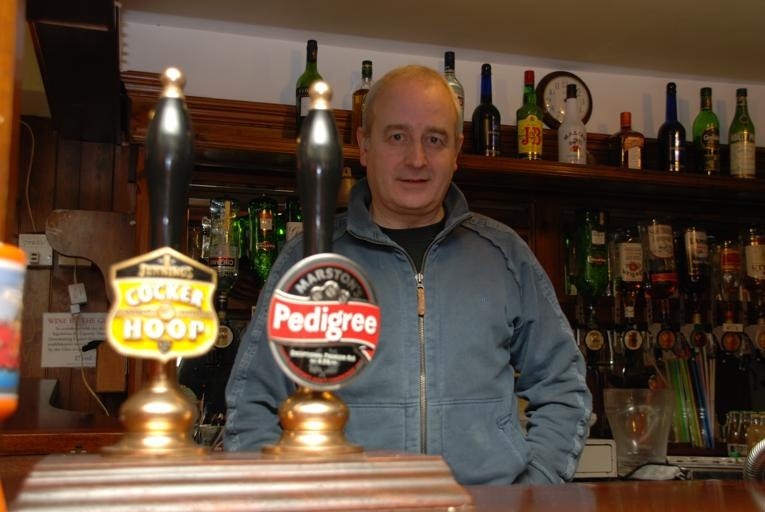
[221,63,595,486]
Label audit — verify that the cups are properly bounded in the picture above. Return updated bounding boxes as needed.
[603,386,675,477]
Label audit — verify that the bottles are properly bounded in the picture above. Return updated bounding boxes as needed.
[439,52,466,132]
[721,409,765,457]
[557,84,588,167]
[191,197,305,325]
[471,63,501,156]
[512,70,544,160]
[294,40,325,137]
[564,207,765,374]
[609,111,646,170]
[349,60,376,144]
[692,87,720,177]
[728,88,757,180]
[658,83,687,173]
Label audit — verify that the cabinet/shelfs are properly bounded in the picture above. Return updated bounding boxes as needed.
[119,130,765,462]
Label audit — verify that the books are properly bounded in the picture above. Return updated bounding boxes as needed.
[666,441,748,481]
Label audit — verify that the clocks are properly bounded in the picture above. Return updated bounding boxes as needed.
[535,70,595,130]
